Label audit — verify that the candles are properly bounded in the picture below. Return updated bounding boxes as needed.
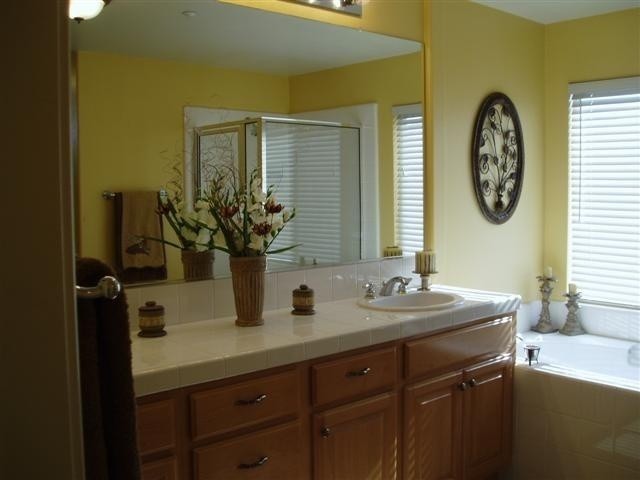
[544,266,552,278]
[568,283,577,295]
[415,249,437,272]
[383,245,402,256]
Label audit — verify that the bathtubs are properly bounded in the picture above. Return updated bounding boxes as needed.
[514,328,640,480]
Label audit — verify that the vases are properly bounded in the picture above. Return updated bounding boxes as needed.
[179,253,214,280]
[228,255,267,328]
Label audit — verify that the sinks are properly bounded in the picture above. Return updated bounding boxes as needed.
[357,289,466,311]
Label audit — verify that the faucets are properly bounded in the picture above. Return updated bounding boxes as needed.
[380,276,409,296]
[516,333,524,341]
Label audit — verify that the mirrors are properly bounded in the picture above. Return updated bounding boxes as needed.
[73,2,426,292]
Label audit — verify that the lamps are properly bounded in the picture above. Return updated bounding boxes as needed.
[66,1,111,24]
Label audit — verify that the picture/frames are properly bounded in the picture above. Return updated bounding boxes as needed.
[470,91,526,226]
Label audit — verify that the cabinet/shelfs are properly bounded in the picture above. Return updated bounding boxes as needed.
[132,388,183,479]
[401,310,517,480]
[309,347,398,480]
[185,365,307,480]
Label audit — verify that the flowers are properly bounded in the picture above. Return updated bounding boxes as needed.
[192,94,303,255]
[140,101,219,250]
